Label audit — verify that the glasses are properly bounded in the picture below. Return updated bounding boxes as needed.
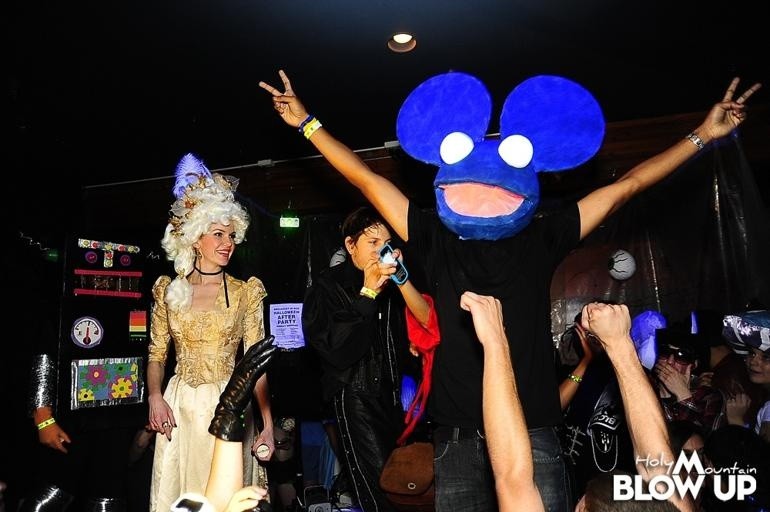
[656,344,693,366]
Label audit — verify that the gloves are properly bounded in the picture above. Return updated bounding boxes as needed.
[208,335,281,444]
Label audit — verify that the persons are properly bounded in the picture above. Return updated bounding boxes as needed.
[137,154,281,510]
[259,69,761,510]
[168,333,282,511]
[13,219,165,511]
[459,288,699,511]
[314,403,347,489]
[302,200,444,511]
[32,336,73,454]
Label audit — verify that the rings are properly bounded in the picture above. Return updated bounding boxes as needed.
[162,420,168,428]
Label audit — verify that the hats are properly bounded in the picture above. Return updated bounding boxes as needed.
[654,321,696,352]
[165,154,250,248]
[722,309,770,356]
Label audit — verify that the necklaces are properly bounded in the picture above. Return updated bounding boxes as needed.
[194,266,224,276]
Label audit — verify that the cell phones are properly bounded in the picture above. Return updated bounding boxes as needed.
[377,245,409,284]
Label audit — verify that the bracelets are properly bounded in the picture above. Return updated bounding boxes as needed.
[297,114,315,133]
[359,285,377,298]
[686,131,704,150]
[37,416,56,431]
[304,121,322,140]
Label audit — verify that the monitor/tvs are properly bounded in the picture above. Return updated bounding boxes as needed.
[278,216,301,230]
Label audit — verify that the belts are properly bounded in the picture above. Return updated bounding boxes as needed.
[438,426,535,440]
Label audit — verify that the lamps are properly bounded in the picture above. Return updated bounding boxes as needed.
[278,186,301,230]
